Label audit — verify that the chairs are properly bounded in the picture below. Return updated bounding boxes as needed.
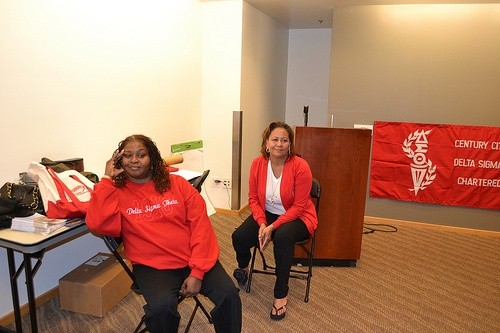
[104,235,214,333]
[246,178,321,303]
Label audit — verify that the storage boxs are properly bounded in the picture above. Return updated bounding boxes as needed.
[58,252,132,317]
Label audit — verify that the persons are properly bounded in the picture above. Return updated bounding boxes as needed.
[85,134,242,333]
[232,121,319,320]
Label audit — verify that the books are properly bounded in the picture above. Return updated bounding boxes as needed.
[11,213,67,234]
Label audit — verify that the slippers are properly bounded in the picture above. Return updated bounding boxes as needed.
[270,298,288,320]
[232,268,248,286]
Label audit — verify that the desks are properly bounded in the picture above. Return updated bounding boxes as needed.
[0,170,205,333]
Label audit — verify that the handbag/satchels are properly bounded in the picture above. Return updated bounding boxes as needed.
[1,181,46,228]
[21,157,100,218]
